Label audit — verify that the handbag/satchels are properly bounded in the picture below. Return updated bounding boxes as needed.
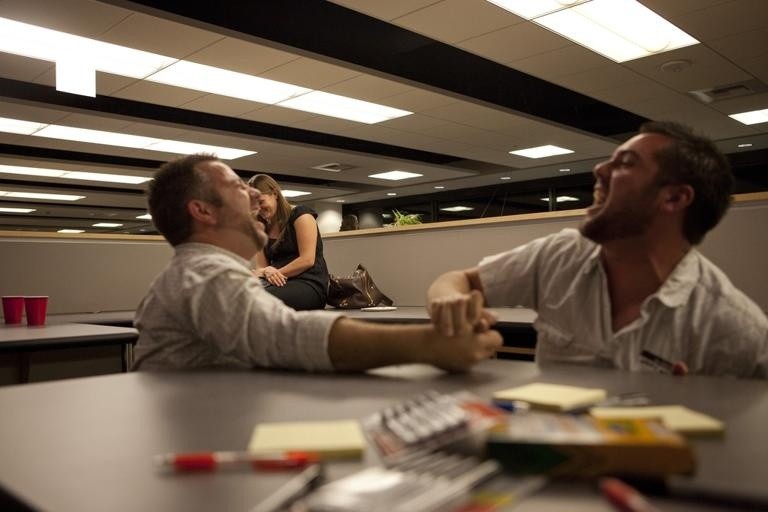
[327,263,393,309]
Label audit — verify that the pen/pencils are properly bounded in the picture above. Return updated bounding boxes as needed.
[163,452,323,512]
[305,390,500,511]
[486,398,531,414]
[599,477,666,512]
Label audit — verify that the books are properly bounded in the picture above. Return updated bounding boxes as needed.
[492,380,610,414]
[361,306,397,311]
[588,402,728,443]
[487,409,701,478]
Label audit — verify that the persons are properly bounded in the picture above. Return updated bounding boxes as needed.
[247,173,330,311]
[423,119,768,379]
[131,152,504,374]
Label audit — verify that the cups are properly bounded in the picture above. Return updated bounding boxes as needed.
[1,296,49,329]
